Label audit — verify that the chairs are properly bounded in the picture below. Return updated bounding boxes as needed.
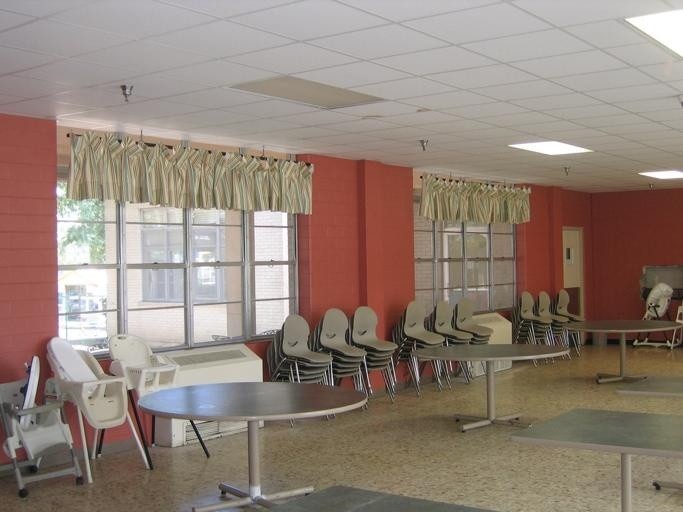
[34,337,150,485]
[96,334,210,470]
[509,289,585,368]
[267,298,493,428]
[0,371,84,497]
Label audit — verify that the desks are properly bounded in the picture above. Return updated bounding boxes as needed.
[413,344,571,433]
[562,319,681,385]
[512,408,683,512]
[271,485,494,512]
[139,381,368,512]
